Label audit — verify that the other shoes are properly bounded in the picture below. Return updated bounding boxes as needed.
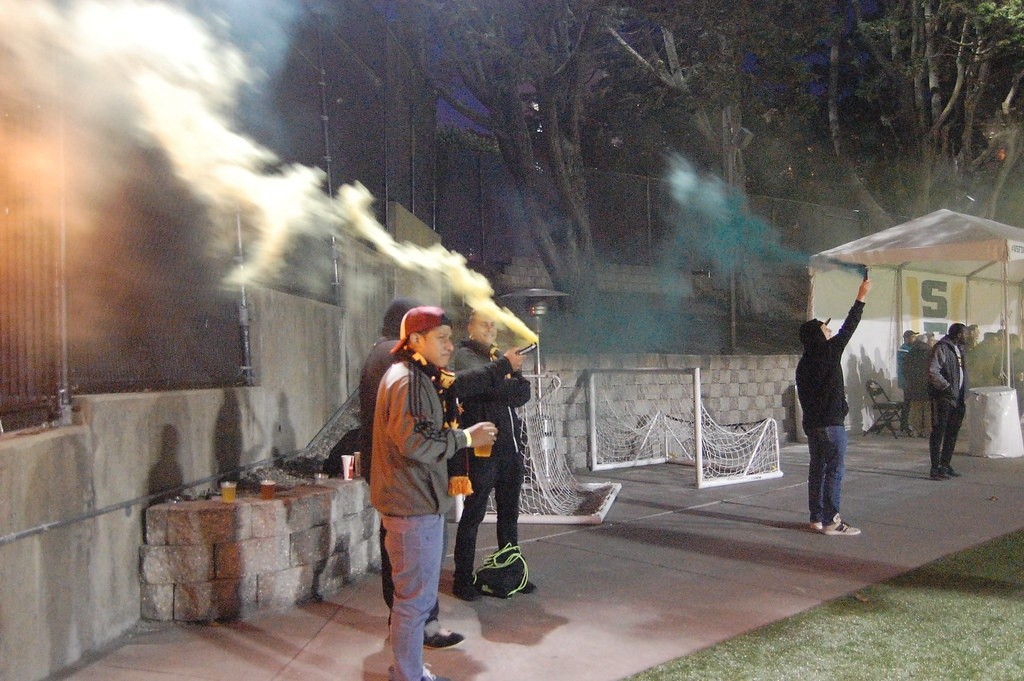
[941,464,963,476]
[516,579,537,593]
[930,468,952,480]
[452,581,480,601]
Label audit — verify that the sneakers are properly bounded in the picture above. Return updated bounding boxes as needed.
[388,663,450,681]
[822,513,861,535]
[423,619,466,649]
[810,520,823,529]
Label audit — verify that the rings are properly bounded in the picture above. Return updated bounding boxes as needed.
[489,431,495,438]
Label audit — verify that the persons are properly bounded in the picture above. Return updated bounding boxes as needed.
[451,307,536,600]
[370,304,498,681]
[796,281,872,536]
[926,324,968,481]
[896,326,1024,437]
[358,298,527,650]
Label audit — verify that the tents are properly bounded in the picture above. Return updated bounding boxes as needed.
[806,207,1024,436]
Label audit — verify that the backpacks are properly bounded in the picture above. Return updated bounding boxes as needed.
[472,542,528,599]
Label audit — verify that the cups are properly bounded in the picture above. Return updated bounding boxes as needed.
[353,452,362,477]
[220,481,237,503]
[474,444,493,457]
[258,480,275,500]
[340,455,355,481]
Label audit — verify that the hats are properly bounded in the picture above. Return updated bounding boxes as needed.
[903,330,919,338]
[390,306,453,355]
[818,318,831,327]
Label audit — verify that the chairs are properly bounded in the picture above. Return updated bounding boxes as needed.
[863,380,914,439]
[616,415,653,462]
